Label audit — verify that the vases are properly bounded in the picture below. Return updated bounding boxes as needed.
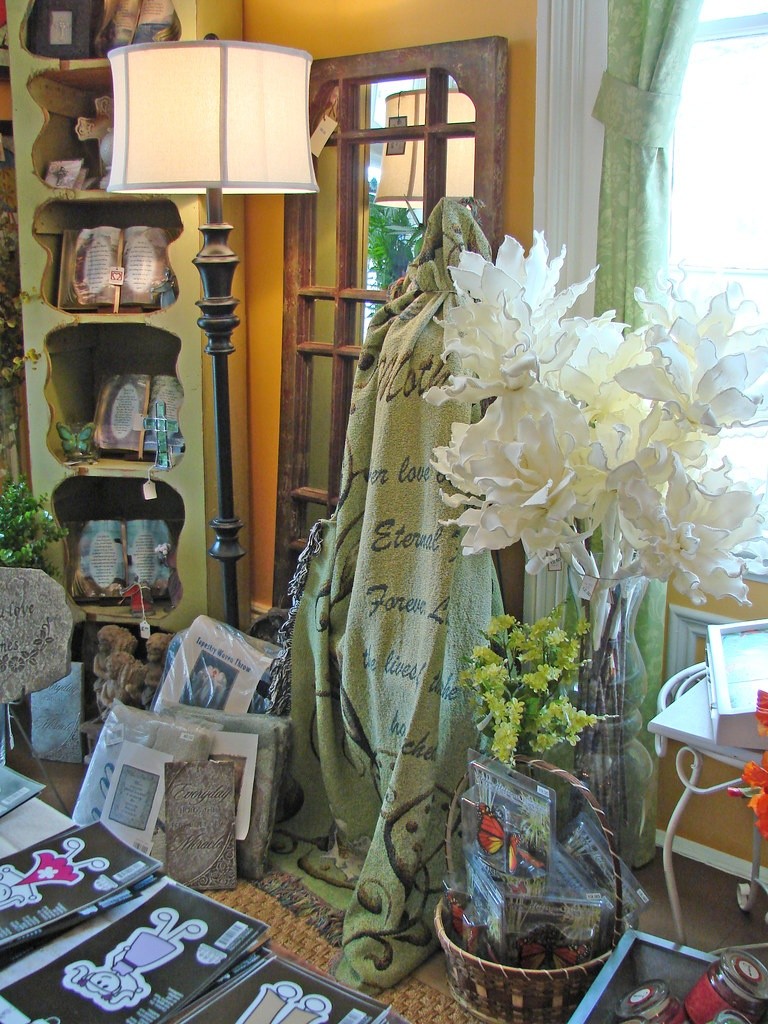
[565,567,647,868]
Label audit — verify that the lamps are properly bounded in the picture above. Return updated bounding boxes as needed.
[107,41,316,625]
[376,91,475,210]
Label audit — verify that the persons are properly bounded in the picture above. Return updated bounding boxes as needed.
[92,625,175,722]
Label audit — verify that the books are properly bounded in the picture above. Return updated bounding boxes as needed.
[64,519,174,601]
[93,373,185,452]
[58,226,172,309]
[95,0,173,58]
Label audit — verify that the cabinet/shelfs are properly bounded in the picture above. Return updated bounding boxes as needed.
[5,1,245,631]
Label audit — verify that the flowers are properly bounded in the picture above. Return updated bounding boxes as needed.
[421,230,767,856]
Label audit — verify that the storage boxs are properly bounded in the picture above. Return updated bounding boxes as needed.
[566,927,721,1024]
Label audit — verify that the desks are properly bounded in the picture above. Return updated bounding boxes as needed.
[649,664,768,946]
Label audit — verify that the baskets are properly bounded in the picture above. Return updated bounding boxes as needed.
[433,753,638,1024]
